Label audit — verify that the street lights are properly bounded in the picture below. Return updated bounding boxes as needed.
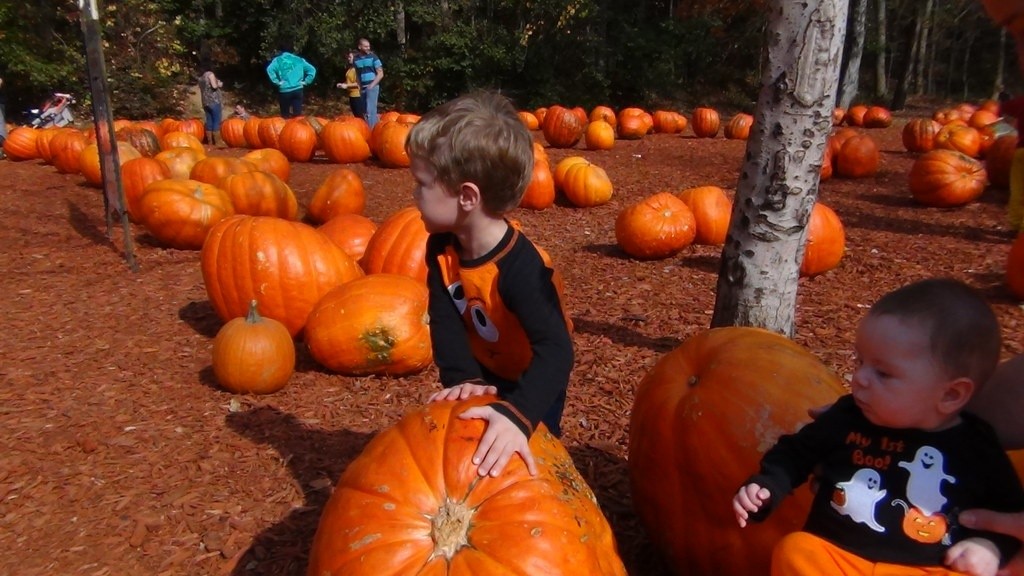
[137,20,167,78]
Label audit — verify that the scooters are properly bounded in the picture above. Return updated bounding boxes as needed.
[21,92,77,129]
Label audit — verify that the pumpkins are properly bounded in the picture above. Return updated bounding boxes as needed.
[797,97,1024,296]
[306,393,636,576]
[629,325,853,576]
[0,105,754,393]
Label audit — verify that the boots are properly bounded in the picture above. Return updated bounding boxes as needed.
[206,131,215,148]
[213,131,227,148]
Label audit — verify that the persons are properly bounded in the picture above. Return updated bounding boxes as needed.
[235,100,250,120]
[337,37,383,127]
[733,277,1024,576]
[266,39,316,119]
[198,58,229,148]
[0,109,8,158]
[1006,108,1024,230]
[405,87,575,477]
